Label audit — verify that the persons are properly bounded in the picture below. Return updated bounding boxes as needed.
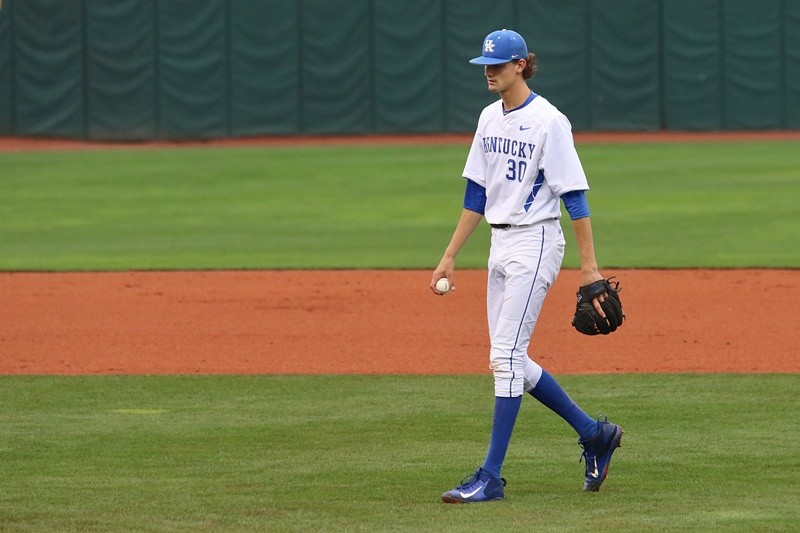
[429,28,624,505]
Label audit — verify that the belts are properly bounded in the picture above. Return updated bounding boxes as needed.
[491,224,509,228]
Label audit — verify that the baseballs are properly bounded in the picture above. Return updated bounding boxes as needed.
[436,277,449,293]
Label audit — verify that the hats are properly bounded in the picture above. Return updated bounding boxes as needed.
[469,29,528,65]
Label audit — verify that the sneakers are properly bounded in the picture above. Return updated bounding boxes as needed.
[441,468,506,502]
[578,415,624,493]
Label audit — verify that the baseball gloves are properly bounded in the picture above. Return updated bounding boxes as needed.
[573,279,623,336]
